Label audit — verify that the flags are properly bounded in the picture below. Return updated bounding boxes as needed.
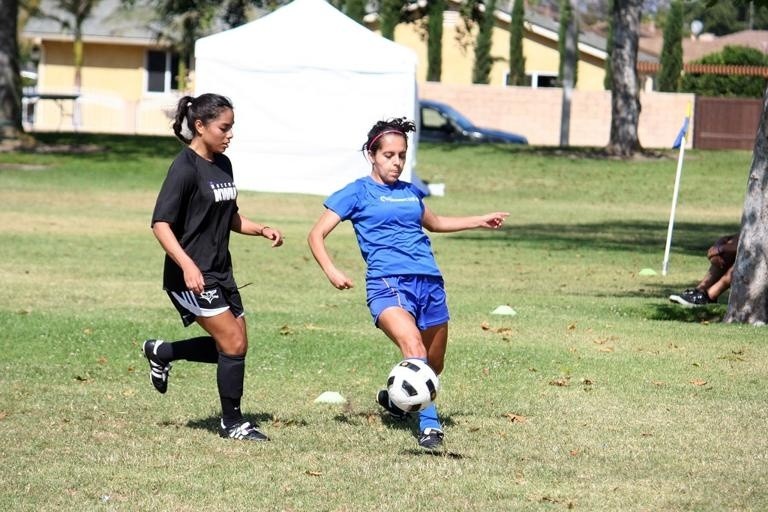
[671,101,693,155]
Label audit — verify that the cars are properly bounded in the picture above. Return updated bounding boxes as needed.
[418,99,528,145]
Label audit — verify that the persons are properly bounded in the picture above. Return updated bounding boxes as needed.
[668,231,740,306]
[140,95,283,442]
[305,115,511,449]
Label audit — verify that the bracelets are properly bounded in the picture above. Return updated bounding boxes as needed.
[260,225,270,236]
[717,245,721,253]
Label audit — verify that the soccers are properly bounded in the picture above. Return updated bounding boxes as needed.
[387,359,439,413]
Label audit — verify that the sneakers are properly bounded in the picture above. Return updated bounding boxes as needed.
[419,428,444,453]
[219,418,271,442]
[143,339,171,393]
[376,391,404,419]
[669,288,717,305]
